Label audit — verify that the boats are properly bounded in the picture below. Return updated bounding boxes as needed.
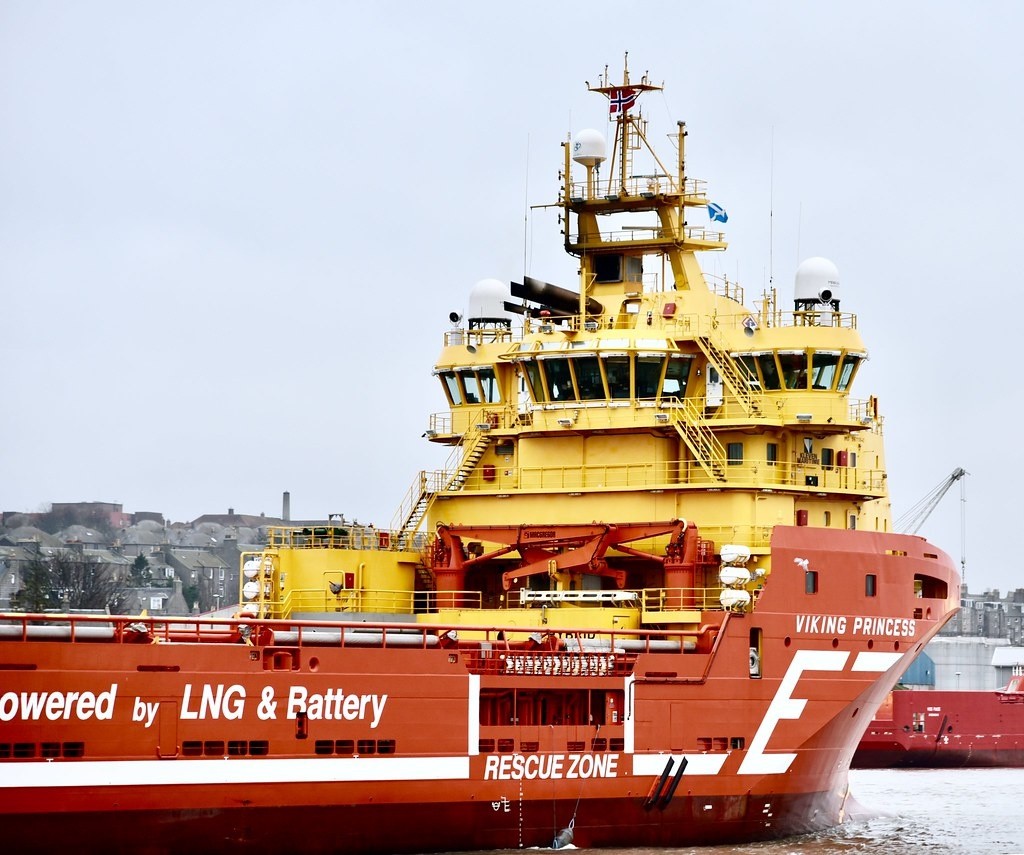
[0,49,1024,855]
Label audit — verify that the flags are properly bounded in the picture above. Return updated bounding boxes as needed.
[708,202,728,224]
[609,89,636,113]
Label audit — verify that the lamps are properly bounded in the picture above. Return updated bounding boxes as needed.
[860,416,873,425]
[583,322,600,331]
[655,414,670,422]
[557,419,574,427]
[796,414,813,423]
[540,325,553,335]
[426,430,437,438]
[476,424,491,431]
